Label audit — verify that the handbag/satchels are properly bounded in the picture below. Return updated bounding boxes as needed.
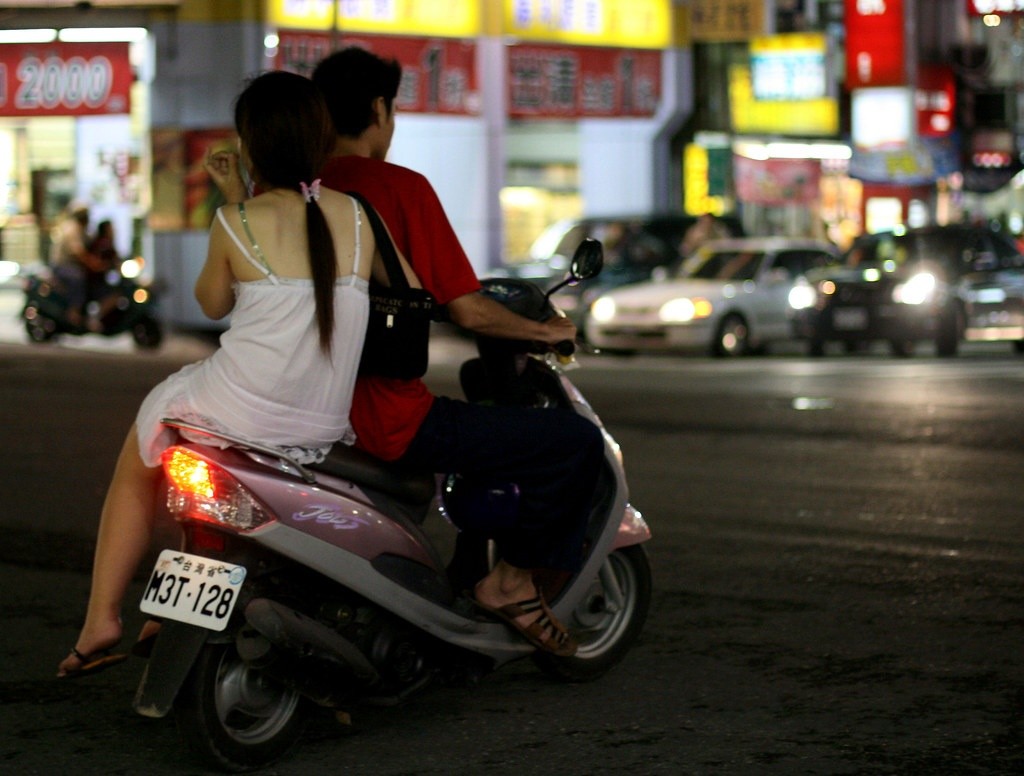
[345,189,434,381]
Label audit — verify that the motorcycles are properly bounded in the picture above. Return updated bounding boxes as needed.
[19,258,165,351]
[144,236,654,773]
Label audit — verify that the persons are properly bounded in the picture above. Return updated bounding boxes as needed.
[253,46,579,658]
[49,210,122,332]
[678,213,728,257]
[56,71,423,680]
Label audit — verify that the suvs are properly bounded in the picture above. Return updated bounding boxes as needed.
[464,208,749,345]
[789,224,1023,358]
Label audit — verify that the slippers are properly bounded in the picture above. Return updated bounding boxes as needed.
[131,616,164,659]
[55,647,128,680]
[469,582,578,658]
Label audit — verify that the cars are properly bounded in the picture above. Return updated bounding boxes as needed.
[584,235,859,360]
